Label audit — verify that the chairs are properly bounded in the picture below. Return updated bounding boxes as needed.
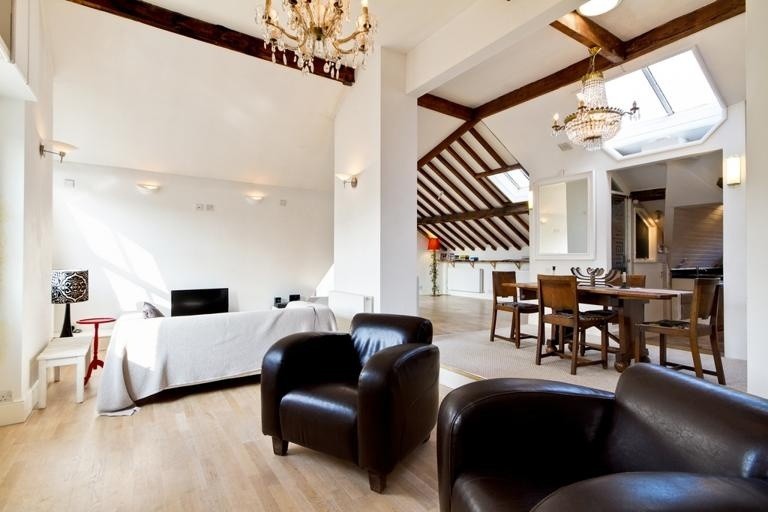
[490,271,730,385]
[262,313,439,493]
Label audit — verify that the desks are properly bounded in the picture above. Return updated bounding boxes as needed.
[76,318,115,385]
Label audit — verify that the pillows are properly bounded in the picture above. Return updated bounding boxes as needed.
[141,303,166,319]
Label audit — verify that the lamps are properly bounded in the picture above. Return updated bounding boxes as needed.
[260,1,376,78]
[40,140,78,163]
[427,237,440,296]
[553,47,640,153]
[726,157,741,185]
[654,209,663,222]
[50,269,88,337]
[335,173,358,188]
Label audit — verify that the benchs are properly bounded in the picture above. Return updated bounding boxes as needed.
[35,336,89,409]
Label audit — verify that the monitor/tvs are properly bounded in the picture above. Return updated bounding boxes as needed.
[171,288,228,317]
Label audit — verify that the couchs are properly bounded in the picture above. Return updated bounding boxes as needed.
[435,363,768,512]
[97,301,336,417]
